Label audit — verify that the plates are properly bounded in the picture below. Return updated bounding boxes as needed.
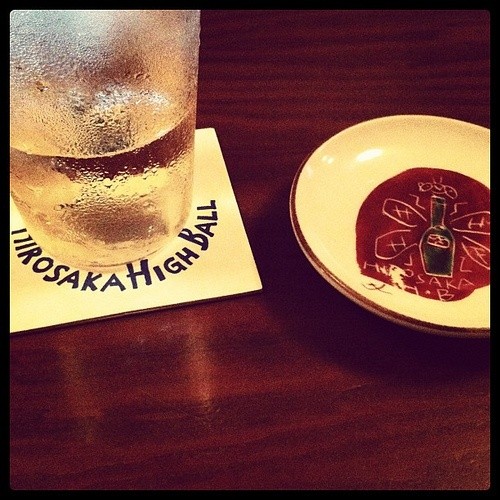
[288,113,489,339]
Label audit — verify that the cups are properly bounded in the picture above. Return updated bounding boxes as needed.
[10,8,199,272]
